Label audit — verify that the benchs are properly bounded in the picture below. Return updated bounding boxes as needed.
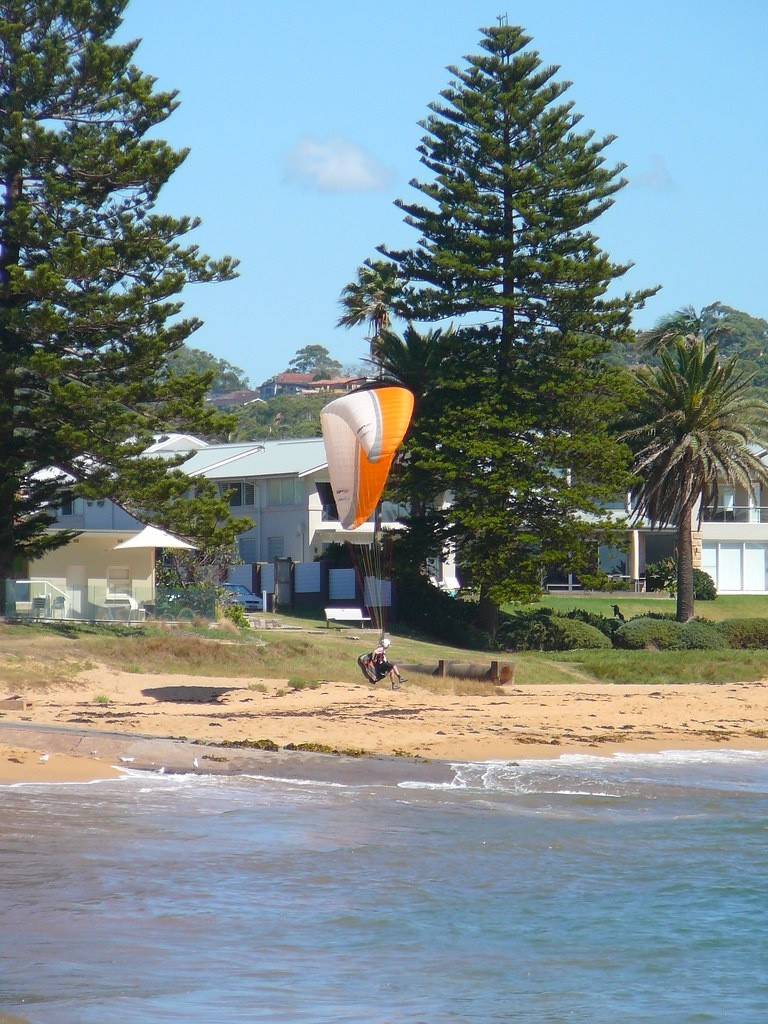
[16,601,45,618]
[324,608,372,629]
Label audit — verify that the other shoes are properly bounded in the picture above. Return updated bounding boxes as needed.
[392,685,401,689]
[399,678,408,684]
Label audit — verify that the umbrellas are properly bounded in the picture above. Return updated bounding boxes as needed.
[106,524,199,600]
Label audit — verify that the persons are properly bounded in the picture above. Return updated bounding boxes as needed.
[372,640,408,690]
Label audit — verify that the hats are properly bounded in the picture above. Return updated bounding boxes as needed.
[380,639,391,648]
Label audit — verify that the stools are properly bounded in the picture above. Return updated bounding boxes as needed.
[134,609,146,621]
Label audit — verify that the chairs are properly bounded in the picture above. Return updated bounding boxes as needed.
[30,593,65,620]
